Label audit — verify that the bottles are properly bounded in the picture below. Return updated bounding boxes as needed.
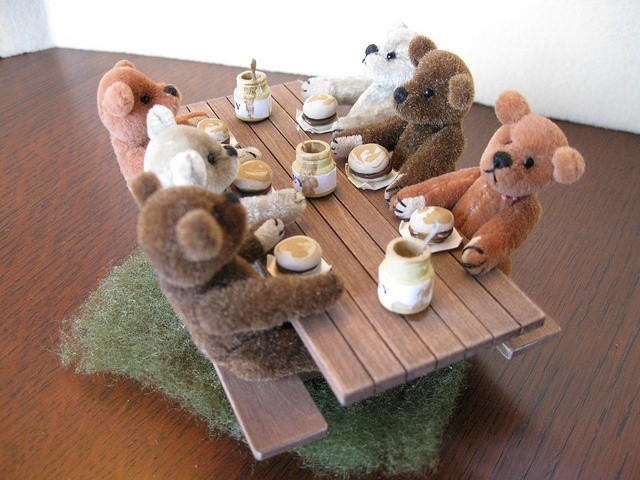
[292,139,337,198]
[377,236,435,315]
[233,70,273,122]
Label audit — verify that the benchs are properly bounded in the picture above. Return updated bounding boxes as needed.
[497,310,561,361]
[212,356,328,463]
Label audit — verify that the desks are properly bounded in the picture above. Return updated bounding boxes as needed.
[163,76,549,412]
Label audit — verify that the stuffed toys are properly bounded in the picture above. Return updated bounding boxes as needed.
[328,35,476,207]
[143,103,308,230]
[96,60,208,188]
[133,171,345,383]
[389,90,586,278]
[302,21,418,134]
